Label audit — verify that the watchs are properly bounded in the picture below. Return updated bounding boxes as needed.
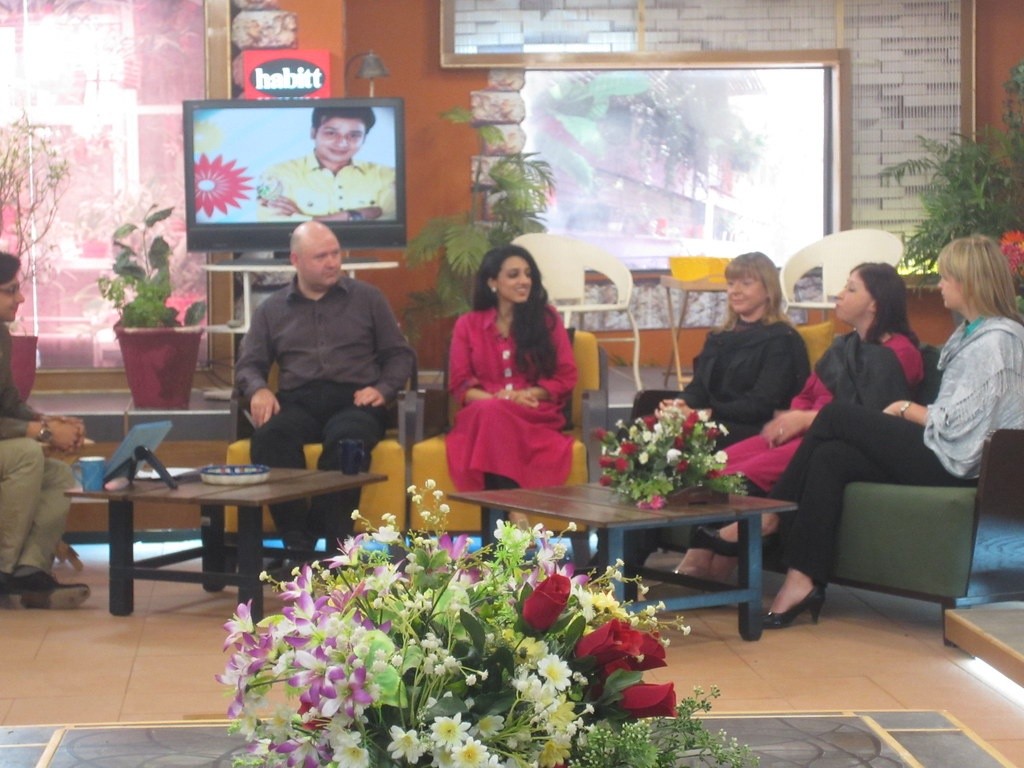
[36,421,53,442]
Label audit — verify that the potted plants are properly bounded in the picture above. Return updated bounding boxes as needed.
[95,204,206,410]
[401,105,556,436]
[0,116,72,402]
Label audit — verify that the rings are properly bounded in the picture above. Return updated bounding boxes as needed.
[505,395,511,399]
[780,427,784,436]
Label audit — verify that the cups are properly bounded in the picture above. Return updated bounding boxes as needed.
[69,456,106,492]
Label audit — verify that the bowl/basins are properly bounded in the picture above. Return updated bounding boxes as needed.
[669,258,733,286]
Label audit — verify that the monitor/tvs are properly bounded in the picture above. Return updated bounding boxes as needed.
[183,96,407,253]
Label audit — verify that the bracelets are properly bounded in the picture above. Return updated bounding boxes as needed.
[346,208,362,222]
[900,400,912,420]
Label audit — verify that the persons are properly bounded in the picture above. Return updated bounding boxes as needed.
[255,105,399,223]
[694,236,1024,631]
[0,252,90,609]
[234,220,417,582]
[592,252,811,607]
[444,246,577,563]
[665,263,923,603]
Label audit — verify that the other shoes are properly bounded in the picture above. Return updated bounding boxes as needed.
[264,545,314,577]
[672,564,710,582]
[0,568,93,610]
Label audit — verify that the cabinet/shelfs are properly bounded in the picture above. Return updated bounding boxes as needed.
[199,255,398,403]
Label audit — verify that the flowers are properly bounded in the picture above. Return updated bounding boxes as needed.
[593,397,749,511]
[212,476,764,767]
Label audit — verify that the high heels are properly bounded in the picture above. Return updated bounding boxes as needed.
[696,524,782,552]
[762,579,824,628]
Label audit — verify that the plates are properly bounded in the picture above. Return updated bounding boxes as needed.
[200,464,271,485]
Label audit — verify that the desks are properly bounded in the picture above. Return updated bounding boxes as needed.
[445,482,800,644]
[62,467,389,626]
[657,275,729,394]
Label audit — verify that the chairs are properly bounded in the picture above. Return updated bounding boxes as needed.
[409,323,611,539]
[511,232,645,394]
[222,337,416,543]
[780,229,905,332]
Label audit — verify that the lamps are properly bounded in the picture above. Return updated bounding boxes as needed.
[344,49,390,98]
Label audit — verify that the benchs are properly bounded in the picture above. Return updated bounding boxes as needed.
[651,341,1024,648]
